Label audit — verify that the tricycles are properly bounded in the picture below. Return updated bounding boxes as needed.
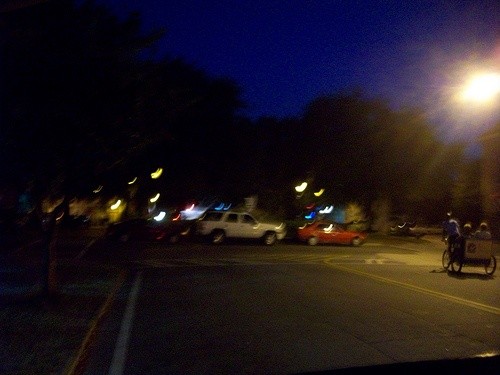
[442,236,497,276]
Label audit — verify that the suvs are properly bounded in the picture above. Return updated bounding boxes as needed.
[195,210,287,247]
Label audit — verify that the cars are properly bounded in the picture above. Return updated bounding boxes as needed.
[105,218,191,245]
[298,220,367,247]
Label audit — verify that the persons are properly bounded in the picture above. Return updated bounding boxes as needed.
[442,212,460,256]
[475,222,491,238]
[455,224,472,249]
